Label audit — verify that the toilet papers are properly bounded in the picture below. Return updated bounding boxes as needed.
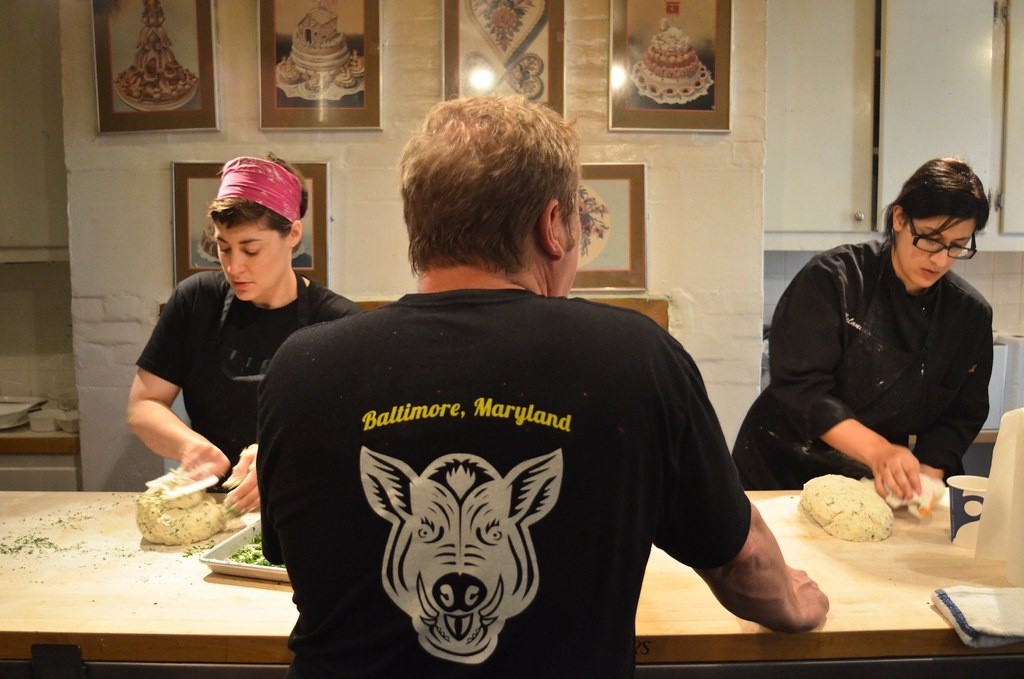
[973,407,1024,588]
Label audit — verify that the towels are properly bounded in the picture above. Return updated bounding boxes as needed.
[930,585,1023,649]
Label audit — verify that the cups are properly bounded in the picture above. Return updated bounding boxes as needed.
[946,474,988,550]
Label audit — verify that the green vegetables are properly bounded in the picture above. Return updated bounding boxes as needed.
[224,530,287,568]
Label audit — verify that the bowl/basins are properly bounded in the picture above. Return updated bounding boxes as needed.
[28,408,64,432]
[55,410,79,433]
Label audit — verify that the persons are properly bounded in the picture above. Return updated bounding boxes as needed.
[255,95,829,679]
[124,157,364,516]
[731,158,994,490]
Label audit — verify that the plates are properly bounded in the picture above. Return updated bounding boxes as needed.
[114,65,201,111]
[198,517,290,582]
[0,396,49,433]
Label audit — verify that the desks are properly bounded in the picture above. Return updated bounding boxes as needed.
[0,492,1024,661]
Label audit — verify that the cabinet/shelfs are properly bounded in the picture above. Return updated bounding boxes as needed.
[763,0,1024,254]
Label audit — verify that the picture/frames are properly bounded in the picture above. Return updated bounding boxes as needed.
[90,0,218,136]
[610,0,732,132]
[568,161,645,291]
[444,0,564,120]
[259,0,382,129]
[170,159,329,299]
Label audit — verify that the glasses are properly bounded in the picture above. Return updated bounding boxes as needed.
[905,215,978,260]
